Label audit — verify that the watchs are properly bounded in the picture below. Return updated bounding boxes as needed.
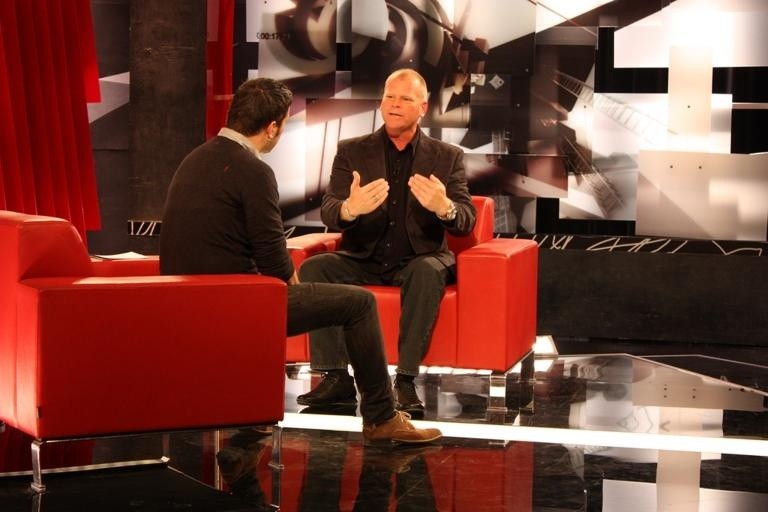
[437,201,457,222]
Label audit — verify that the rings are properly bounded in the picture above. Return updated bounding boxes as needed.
[371,196,379,204]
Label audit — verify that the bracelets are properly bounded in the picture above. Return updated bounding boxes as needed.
[342,198,356,220]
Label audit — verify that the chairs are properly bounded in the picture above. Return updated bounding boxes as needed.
[277,187,543,412]
[1,205,289,489]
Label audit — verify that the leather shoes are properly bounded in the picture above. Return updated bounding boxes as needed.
[361,408,442,450]
[392,376,426,417]
[296,372,360,407]
[236,425,273,437]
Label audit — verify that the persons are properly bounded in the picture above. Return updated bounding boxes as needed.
[297,68,476,416]
[215,425,442,512]
[159,78,443,443]
